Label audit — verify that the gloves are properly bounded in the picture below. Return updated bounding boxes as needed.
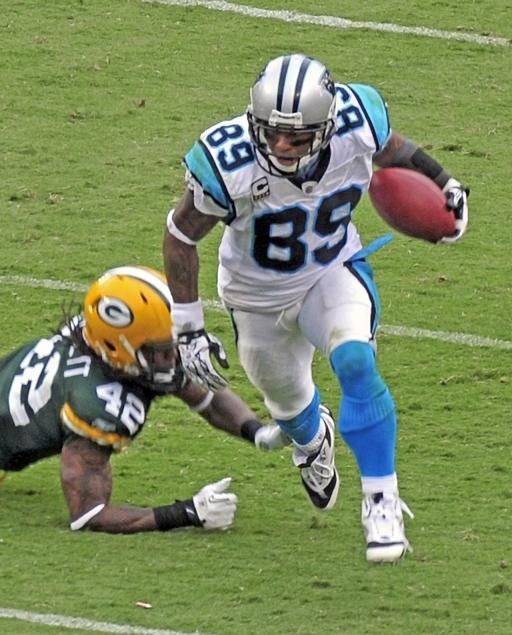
[175,327,229,395]
[191,477,238,530]
[440,176,468,242]
[255,424,292,453]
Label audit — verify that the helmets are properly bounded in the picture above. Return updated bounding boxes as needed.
[247,54,336,180]
[83,264,191,394]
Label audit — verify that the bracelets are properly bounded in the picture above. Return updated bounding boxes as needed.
[410,144,443,184]
[241,421,260,443]
[153,503,191,533]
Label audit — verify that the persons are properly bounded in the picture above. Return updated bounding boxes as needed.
[1,263,331,533]
[161,51,470,565]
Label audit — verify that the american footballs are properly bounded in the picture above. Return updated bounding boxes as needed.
[369,168,455,242]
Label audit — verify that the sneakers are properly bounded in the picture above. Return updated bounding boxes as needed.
[361,491,415,563]
[293,404,341,510]
